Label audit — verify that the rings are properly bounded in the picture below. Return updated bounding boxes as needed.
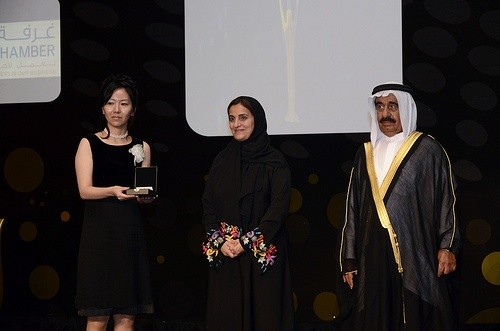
[344,273,349,275]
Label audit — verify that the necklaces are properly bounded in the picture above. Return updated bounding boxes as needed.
[104,128,128,138]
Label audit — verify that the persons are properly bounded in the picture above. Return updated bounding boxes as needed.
[202,96,291,331]
[75,74,158,331]
[339,82,462,331]
